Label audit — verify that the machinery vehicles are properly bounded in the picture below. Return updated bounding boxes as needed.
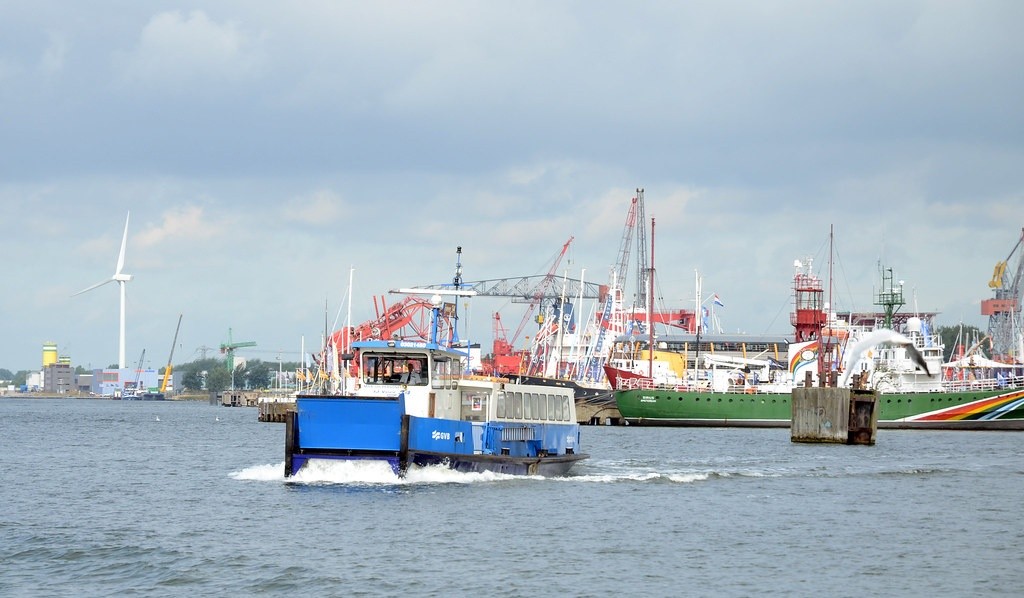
[144,313,183,398]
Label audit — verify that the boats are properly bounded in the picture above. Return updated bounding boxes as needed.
[252,186,1024,475]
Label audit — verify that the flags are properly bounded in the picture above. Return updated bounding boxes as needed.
[319,371,329,380]
[298,369,306,380]
[308,370,314,381]
[714,295,723,307]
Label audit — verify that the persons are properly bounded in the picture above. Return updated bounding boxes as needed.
[1006,371,1018,389]
[399,363,421,386]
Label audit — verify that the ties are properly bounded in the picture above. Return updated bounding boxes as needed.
[407,372,411,384]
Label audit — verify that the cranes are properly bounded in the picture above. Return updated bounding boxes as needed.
[221,327,257,377]
